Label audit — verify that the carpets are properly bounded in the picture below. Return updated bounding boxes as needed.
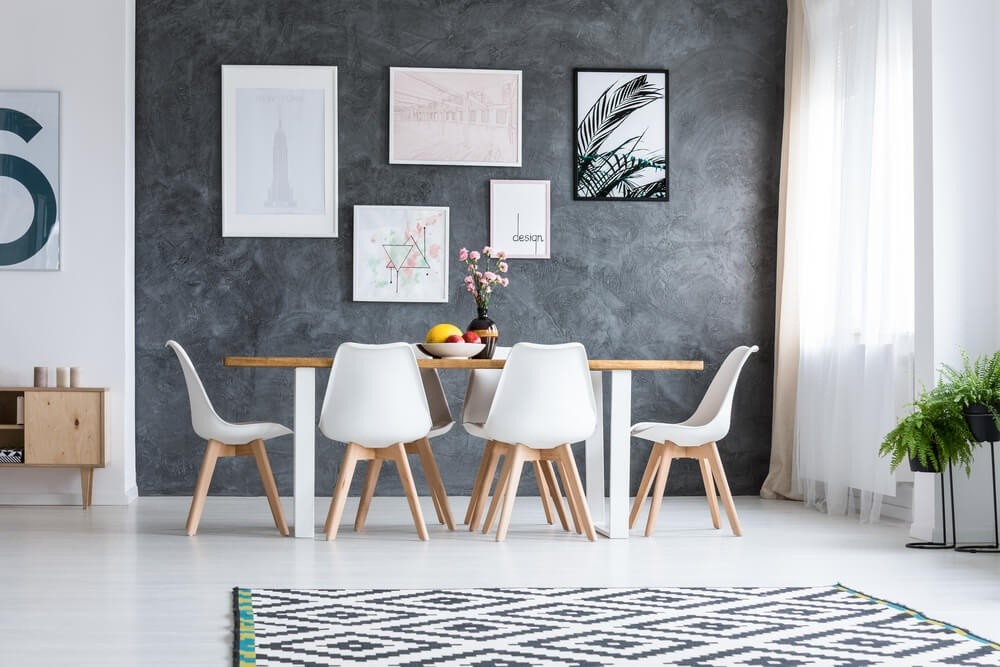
[230,583,1000,667]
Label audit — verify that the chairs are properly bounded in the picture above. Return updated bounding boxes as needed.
[628,345,760,537]
[318,342,601,542]
[165,339,291,538]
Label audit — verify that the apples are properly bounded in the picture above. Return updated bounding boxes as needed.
[445,332,481,343]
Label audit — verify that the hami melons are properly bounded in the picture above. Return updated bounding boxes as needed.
[425,324,463,342]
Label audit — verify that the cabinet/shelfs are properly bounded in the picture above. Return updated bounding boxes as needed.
[0,385,107,512]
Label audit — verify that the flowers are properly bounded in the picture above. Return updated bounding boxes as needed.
[458,246,509,306]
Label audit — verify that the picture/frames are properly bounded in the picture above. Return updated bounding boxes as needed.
[220,64,670,302]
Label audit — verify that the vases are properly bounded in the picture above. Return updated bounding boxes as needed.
[467,307,499,360]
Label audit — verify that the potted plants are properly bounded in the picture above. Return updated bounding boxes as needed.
[878,350,1000,477]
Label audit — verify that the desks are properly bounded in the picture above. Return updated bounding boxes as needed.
[223,356,703,538]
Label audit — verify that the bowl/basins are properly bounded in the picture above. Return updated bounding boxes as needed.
[421,343,487,360]
[416,343,441,359]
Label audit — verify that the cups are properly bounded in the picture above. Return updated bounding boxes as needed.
[70,366,81,387]
[34,367,48,387]
[56,367,69,387]
